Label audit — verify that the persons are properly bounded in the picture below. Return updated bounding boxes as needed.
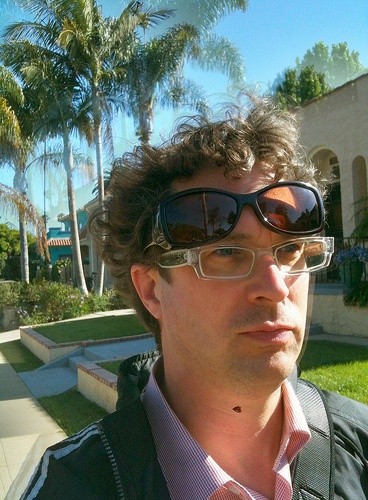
[14,93,366,500]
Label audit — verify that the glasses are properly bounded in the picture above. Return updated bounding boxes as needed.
[142,180,329,255]
[153,235,334,281]
